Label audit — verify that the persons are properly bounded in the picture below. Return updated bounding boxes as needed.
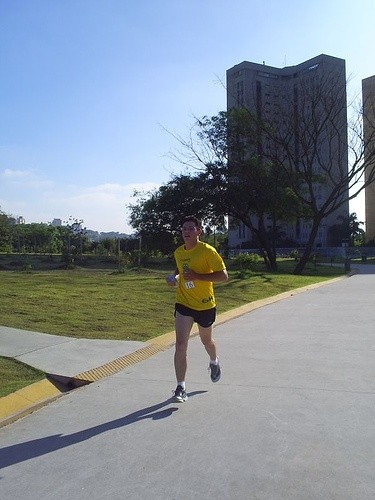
[166,215,229,403]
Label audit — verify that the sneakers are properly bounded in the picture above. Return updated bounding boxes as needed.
[174,385,188,402]
[209,356,222,383]
